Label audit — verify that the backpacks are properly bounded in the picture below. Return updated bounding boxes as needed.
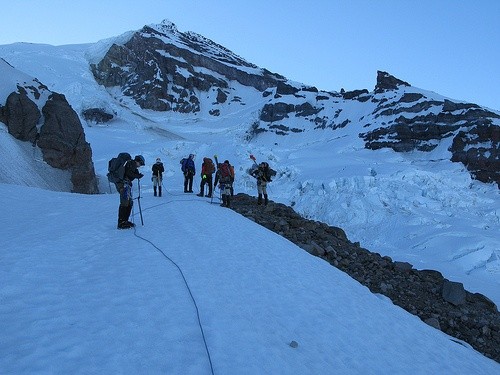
[180,158,190,176]
[218,163,232,183]
[205,158,215,173]
[106,152,132,183]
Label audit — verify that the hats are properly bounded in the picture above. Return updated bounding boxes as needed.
[135,155,145,166]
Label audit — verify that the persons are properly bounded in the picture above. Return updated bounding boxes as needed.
[114,155,145,229]
[257,162,269,205]
[183,154,196,193]
[214,160,234,208]
[151,158,164,197]
[196,157,212,198]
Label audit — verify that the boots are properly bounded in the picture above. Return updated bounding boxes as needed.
[117,201,136,228]
[257,194,269,206]
[220,194,230,207]
[158,185,161,197]
[154,186,157,196]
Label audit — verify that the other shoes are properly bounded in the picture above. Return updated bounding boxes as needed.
[184,191,188,193]
[189,191,193,193]
[204,193,211,198]
[196,192,204,197]
